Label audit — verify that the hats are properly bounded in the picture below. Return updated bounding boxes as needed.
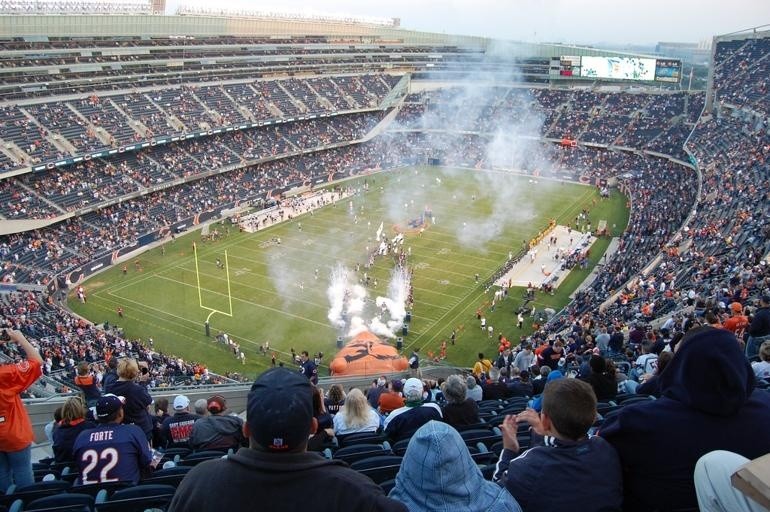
[404,378,423,397]
[96,394,126,418]
[208,395,224,413]
[174,395,189,410]
[195,399,208,412]
[247,367,312,451]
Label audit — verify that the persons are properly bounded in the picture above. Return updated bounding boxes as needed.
[1,37,769,511]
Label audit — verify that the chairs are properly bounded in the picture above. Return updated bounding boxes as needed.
[0,36,385,279]
[1,278,235,397]
[495,34,769,510]
[386,37,702,187]
[1,364,521,507]
